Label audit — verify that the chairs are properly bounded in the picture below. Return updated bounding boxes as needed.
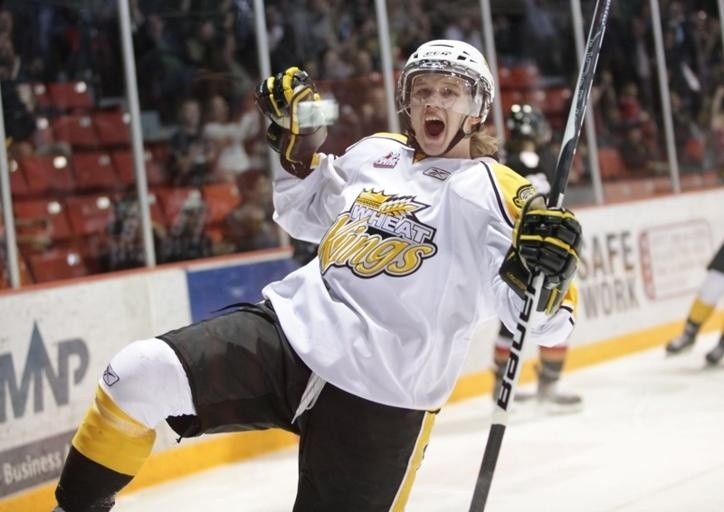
[0,0,723,290]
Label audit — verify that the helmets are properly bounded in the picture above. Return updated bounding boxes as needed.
[506,104,554,144]
[395,40,496,124]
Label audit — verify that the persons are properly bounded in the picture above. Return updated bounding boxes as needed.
[489,99,581,407]
[1,0,724,274]
[666,242,724,363]
[51,38,582,512]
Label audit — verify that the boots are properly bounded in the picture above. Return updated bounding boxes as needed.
[495,361,579,403]
[667,318,724,364]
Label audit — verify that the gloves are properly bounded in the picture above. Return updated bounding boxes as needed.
[255,68,329,179]
[499,193,583,310]
